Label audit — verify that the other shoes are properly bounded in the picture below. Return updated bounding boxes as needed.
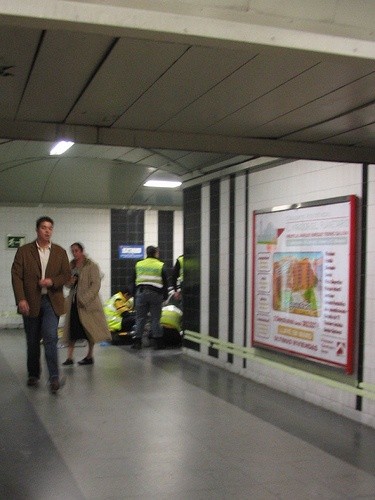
[50,380,58,393]
[62,359,74,365]
[28,377,38,386]
[78,357,93,365]
[132,341,141,349]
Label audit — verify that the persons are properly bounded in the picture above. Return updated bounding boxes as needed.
[60,241,112,366]
[103,245,184,351]
[11,216,72,393]
